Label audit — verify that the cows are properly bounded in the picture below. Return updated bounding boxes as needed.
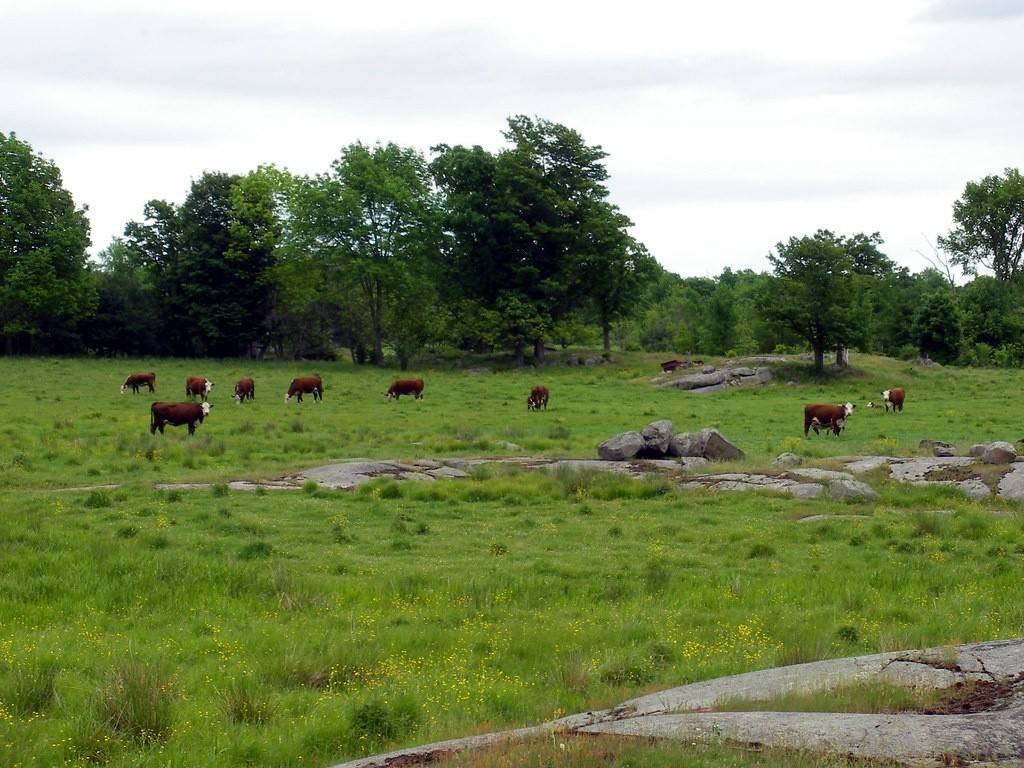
[527,385,550,412]
[150,401,215,437]
[384,378,424,403]
[231,377,256,407]
[661,360,704,375]
[866,402,884,410]
[283,376,324,406]
[120,372,157,396]
[804,401,857,439]
[879,387,906,415]
[185,377,216,402]
